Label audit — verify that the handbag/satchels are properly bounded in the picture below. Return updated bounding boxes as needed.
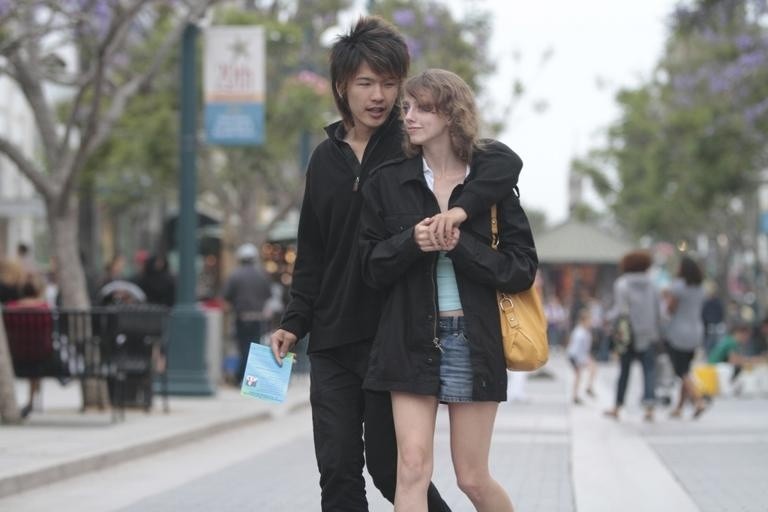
[689,360,720,399]
[496,282,548,371]
[614,317,633,357]
[702,297,725,325]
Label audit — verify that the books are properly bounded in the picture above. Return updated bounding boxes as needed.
[241,340,297,404]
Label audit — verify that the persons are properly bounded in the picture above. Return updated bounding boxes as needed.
[1,232,309,384]
[348,66,541,512]
[272,15,525,510]
[528,241,768,418]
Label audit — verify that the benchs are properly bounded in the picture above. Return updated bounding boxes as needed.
[2,304,167,418]
